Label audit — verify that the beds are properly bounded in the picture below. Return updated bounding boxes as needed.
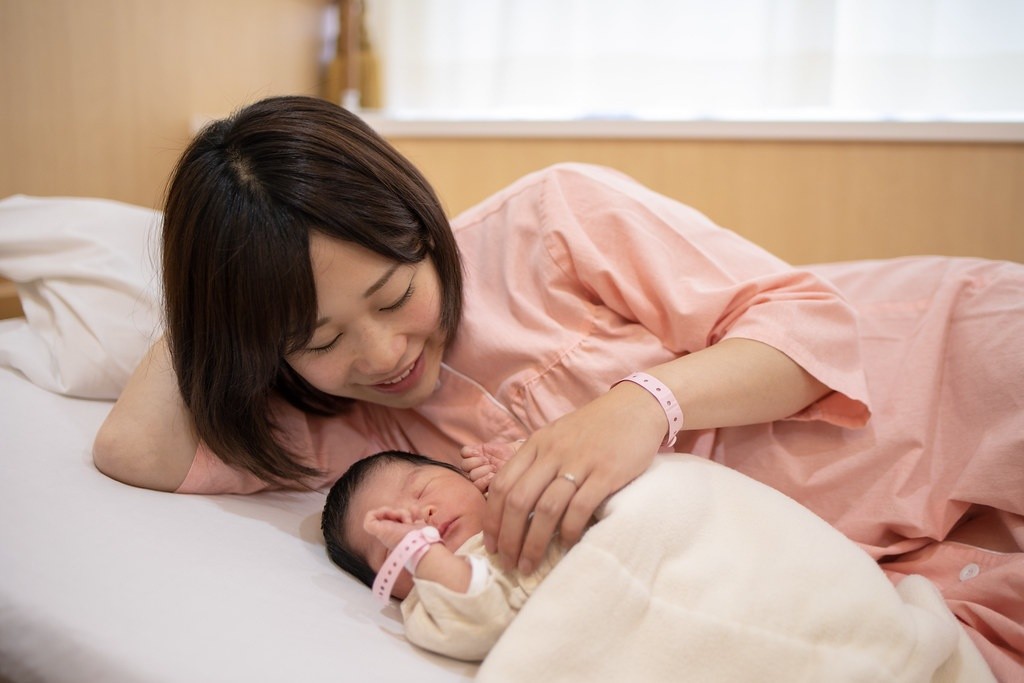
[0,364,479,683]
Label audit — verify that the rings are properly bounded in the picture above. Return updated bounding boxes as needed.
[557,473,579,489]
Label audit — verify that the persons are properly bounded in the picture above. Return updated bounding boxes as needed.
[322,439,599,664]
[93,95,1024,683]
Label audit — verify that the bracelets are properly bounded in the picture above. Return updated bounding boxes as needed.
[373,527,445,612]
[610,372,683,448]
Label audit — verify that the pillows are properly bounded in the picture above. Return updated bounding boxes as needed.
[0,191,165,399]
